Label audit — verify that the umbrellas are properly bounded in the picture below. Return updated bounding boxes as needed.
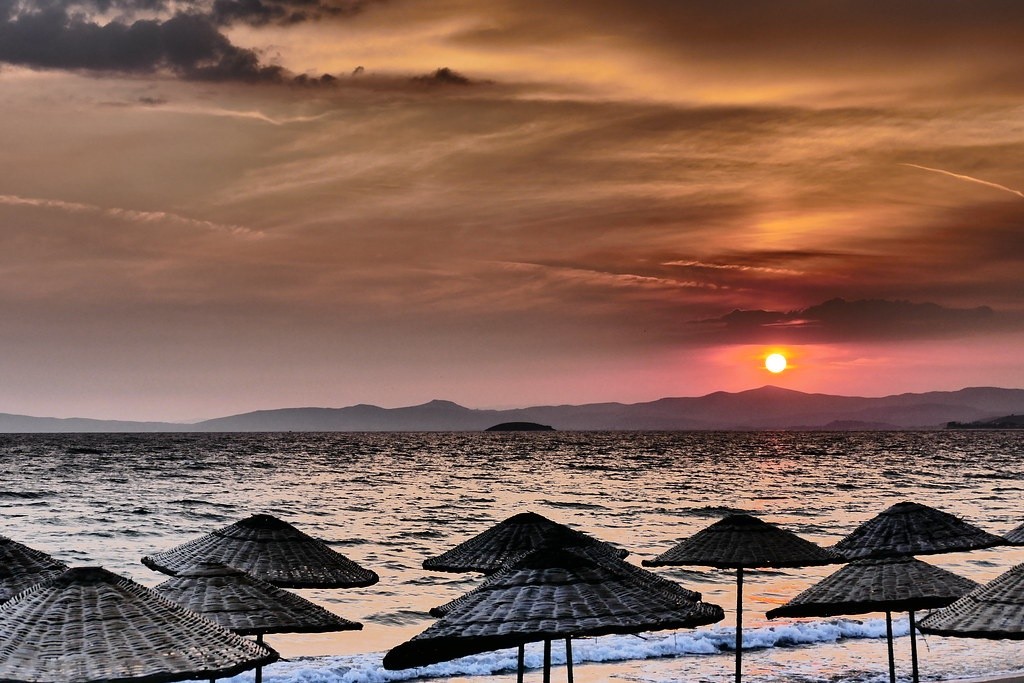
[766,502,1013,683]
[916,563,1024,640]
[1002,522,1024,545]
[383,513,725,683]
[150,563,364,683]
[642,514,845,683]
[0,534,278,683]
[141,514,379,589]
